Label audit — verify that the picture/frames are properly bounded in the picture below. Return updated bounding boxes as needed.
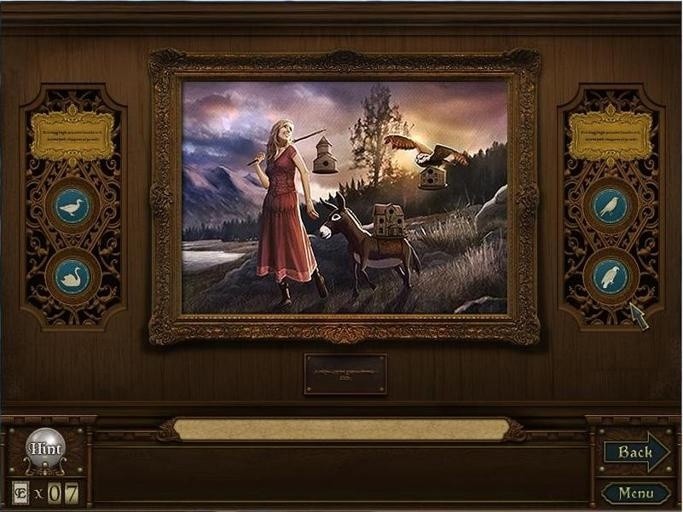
[146,46,543,351]
